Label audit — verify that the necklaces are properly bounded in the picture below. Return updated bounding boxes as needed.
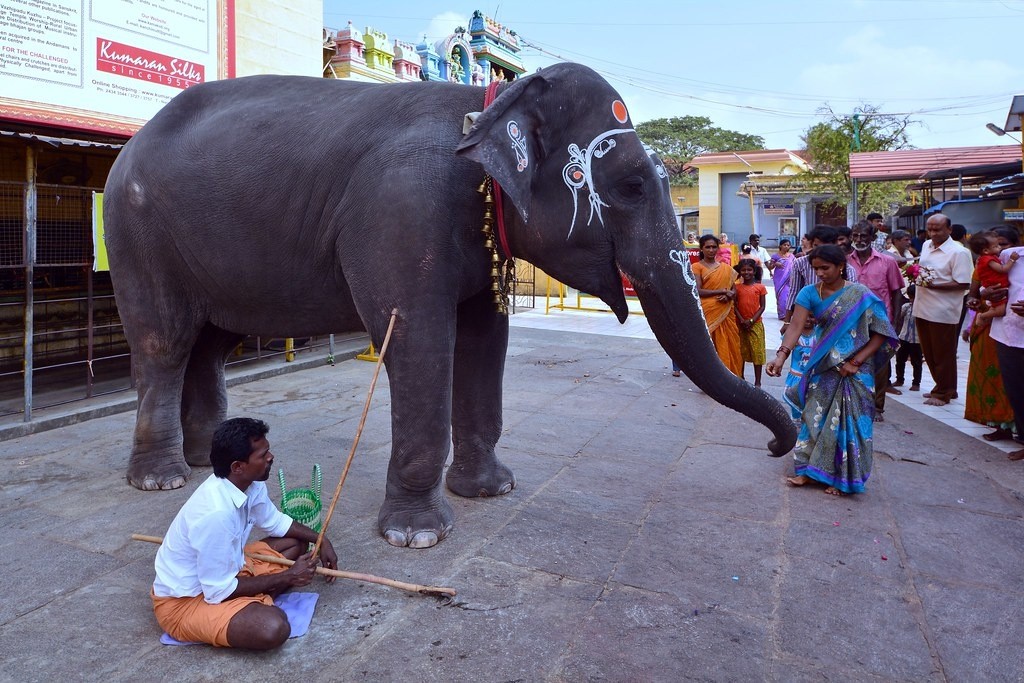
[780,251,787,256]
[820,282,823,301]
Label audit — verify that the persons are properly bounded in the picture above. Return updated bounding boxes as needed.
[150,418,339,648]
[672,233,772,388]
[767,211,974,422]
[764,245,897,495]
[963,226,1024,461]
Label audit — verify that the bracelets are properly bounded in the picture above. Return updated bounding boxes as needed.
[776,346,791,360]
[1010,258,1015,262]
[750,318,754,325]
[850,357,860,367]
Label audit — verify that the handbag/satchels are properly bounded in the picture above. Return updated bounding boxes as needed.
[278,463,322,552]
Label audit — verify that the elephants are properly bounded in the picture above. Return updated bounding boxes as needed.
[102,62,798,549]
